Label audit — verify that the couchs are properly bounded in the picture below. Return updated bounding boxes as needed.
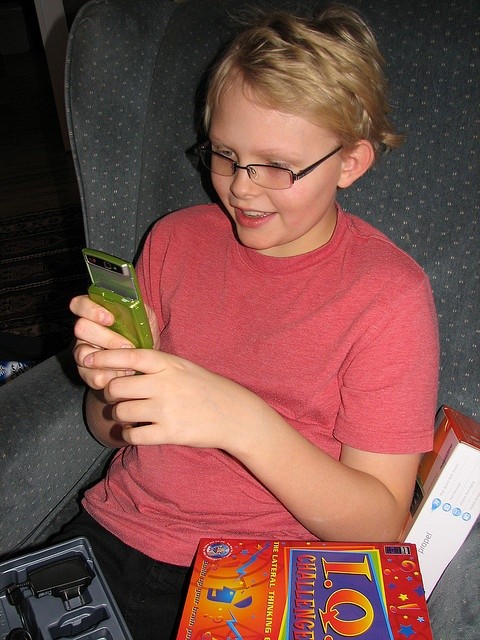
[0,2,480,640]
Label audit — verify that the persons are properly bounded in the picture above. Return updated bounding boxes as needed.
[37,0,441,640]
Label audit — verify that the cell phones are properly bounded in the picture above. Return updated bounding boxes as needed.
[79,247,153,352]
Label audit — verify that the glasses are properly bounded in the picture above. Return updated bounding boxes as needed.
[194,141,343,190]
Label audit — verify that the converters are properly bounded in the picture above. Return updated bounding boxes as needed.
[15,554,100,608]
